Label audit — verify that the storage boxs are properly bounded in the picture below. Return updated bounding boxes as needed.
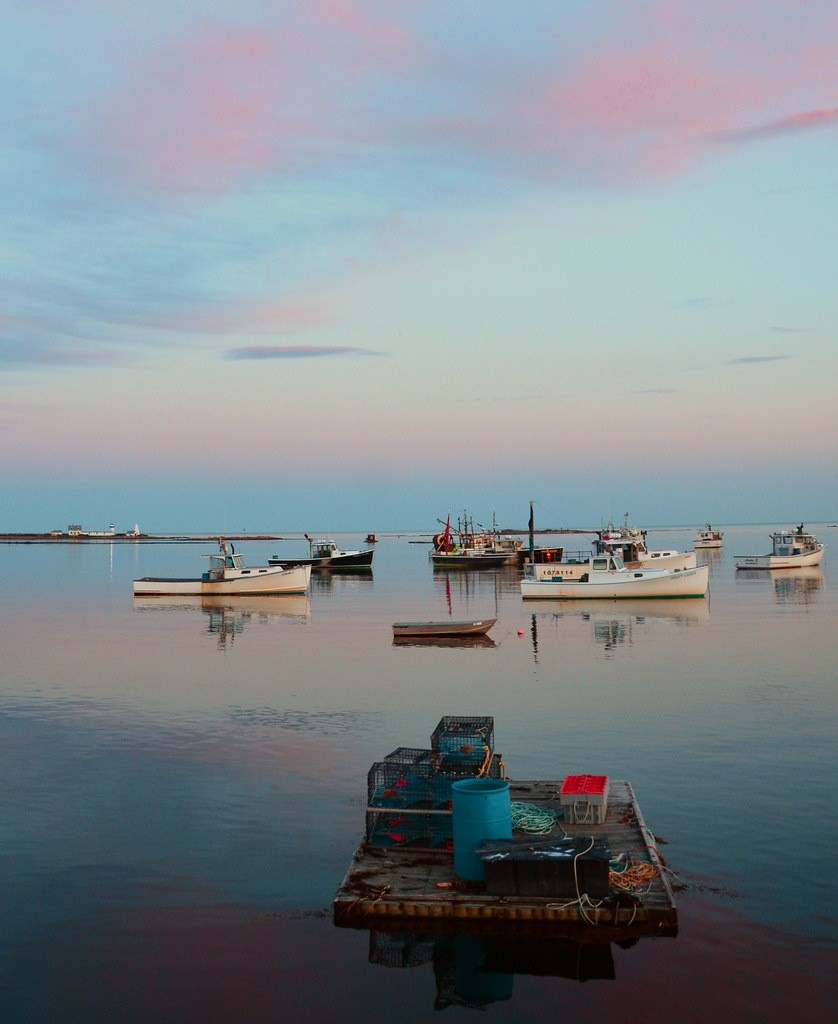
[560,774,609,825]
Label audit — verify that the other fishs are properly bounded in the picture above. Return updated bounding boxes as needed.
[384,777,408,798]
[387,818,406,844]
[459,744,474,755]
[446,839,455,852]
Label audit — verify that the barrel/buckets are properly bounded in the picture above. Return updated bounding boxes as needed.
[202,573,210,581]
[273,555,278,559]
[451,779,513,879]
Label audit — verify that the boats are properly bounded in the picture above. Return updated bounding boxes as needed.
[391,618,498,636]
[522,538,697,582]
[732,522,829,570]
[267,533,375,569]
[519,555,710,599]
[591,529,678,563]
[366,534,376,543]
[427,504,518,571]
[693,521,725,548]
[132,536,313,595]
[332,715,679,939]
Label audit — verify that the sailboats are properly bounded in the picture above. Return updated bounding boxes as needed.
[457,509,524,548]
